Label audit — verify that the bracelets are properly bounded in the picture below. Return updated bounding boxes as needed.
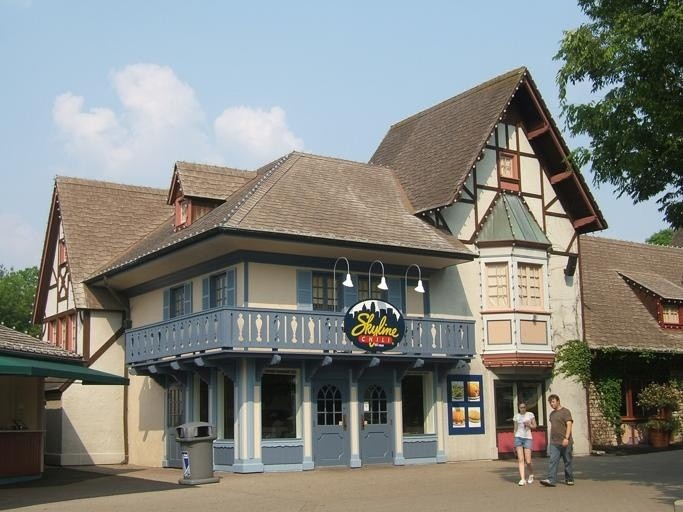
[565,436,569,440]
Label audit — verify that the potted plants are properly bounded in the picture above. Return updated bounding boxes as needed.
[640,417,682,448]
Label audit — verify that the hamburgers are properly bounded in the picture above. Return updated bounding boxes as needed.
[468,410,479,423]
[452,410,465,426]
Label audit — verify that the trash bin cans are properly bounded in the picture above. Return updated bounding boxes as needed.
[175,421,220,485]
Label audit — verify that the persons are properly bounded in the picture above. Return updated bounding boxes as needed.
[539,394,575,487]
[512,400,537,486]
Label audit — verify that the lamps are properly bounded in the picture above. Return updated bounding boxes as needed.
[333,255,354,312]
[404,264,425,314]
[368,260,388,299]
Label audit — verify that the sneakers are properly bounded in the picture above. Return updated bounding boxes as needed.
[528,474,533,484]
[519,480,526,486]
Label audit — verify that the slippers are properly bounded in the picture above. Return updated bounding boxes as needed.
[566,480,574,485]
[540,479,556,487]
